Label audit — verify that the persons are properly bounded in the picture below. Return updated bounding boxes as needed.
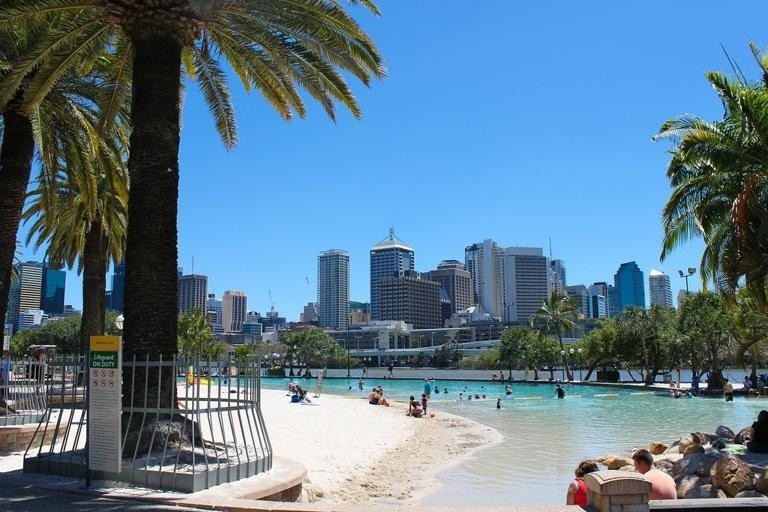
[566,461,600,507]
[666,368,768,402]
[745,410,768,454]
[632,449,678,500]
[552,383,566,399]
[344,363,512,418]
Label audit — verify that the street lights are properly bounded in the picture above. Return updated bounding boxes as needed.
[578,348,582,381]
[347,315,350,378]
[560,351,565,381]
[570,349,574,381]
